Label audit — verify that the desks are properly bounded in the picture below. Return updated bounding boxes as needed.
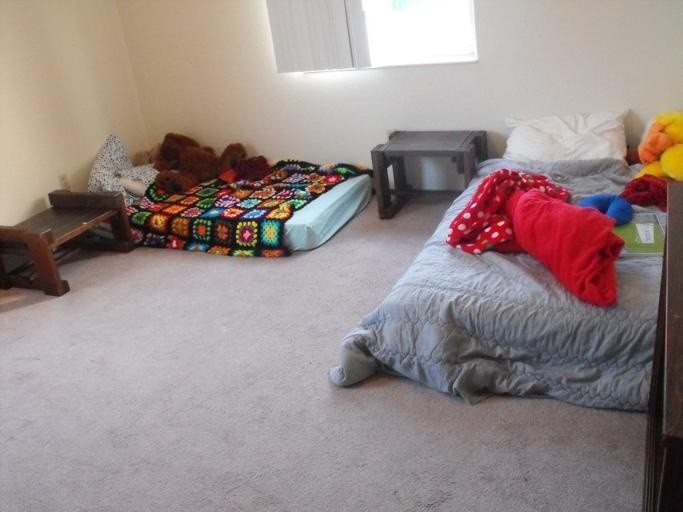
[1,189,135,296]
[642,180,682,511]
[370,129,488,220]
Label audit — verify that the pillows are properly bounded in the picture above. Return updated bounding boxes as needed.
[500,107,631,168]
[88,133,160,206]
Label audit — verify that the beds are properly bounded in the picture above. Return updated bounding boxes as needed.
[85,164,374,252]
[383,155,666,393]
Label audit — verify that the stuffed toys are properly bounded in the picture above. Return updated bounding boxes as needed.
[130,132,247,194]
[633,112,683,182]
